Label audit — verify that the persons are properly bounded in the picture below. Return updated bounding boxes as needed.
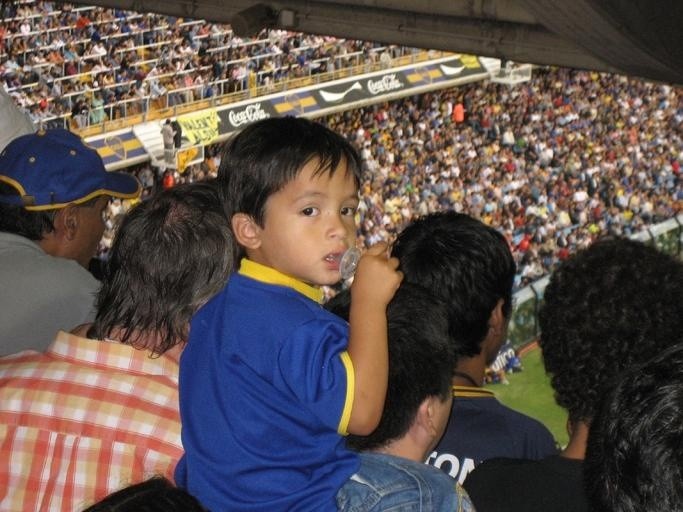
[0,128,141,362]
[311,64,683,304]
[174,114,560,510]
[464,231,682,512]
[160,117,181,164]
[1,177,243,511]
[92,141,226,258]
[1,0,433,131]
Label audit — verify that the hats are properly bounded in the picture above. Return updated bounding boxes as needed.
[0,128,141,213]
